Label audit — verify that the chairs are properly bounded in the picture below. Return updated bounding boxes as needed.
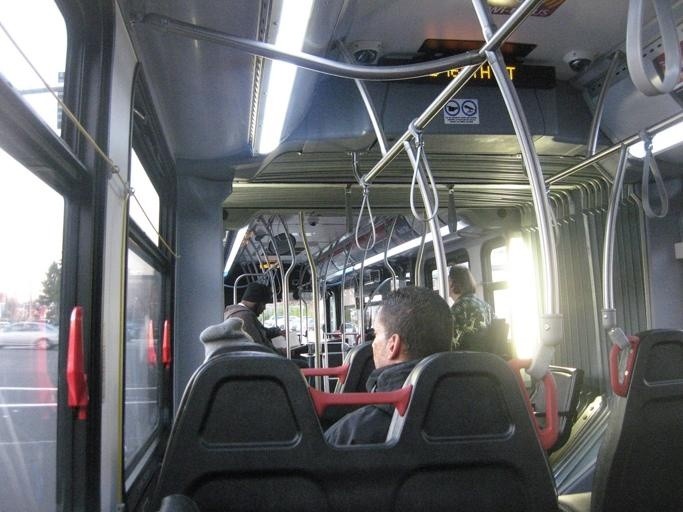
[152,328,683,512]
[0,393,40,512]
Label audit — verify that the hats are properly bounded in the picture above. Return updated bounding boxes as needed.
[240,282,272,303]
[199,316,256,362]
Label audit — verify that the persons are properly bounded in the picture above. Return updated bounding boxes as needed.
[318,285,454,446]
[194,316,257,366]
[445,265,497,351]
[222,281,311,384]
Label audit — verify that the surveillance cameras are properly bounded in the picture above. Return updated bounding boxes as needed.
[561,50,595,73]
[352,40,381,64]
[308,217,320,227]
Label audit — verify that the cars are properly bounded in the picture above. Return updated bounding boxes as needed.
[265,314,356,344]
[0,322,59,350]
